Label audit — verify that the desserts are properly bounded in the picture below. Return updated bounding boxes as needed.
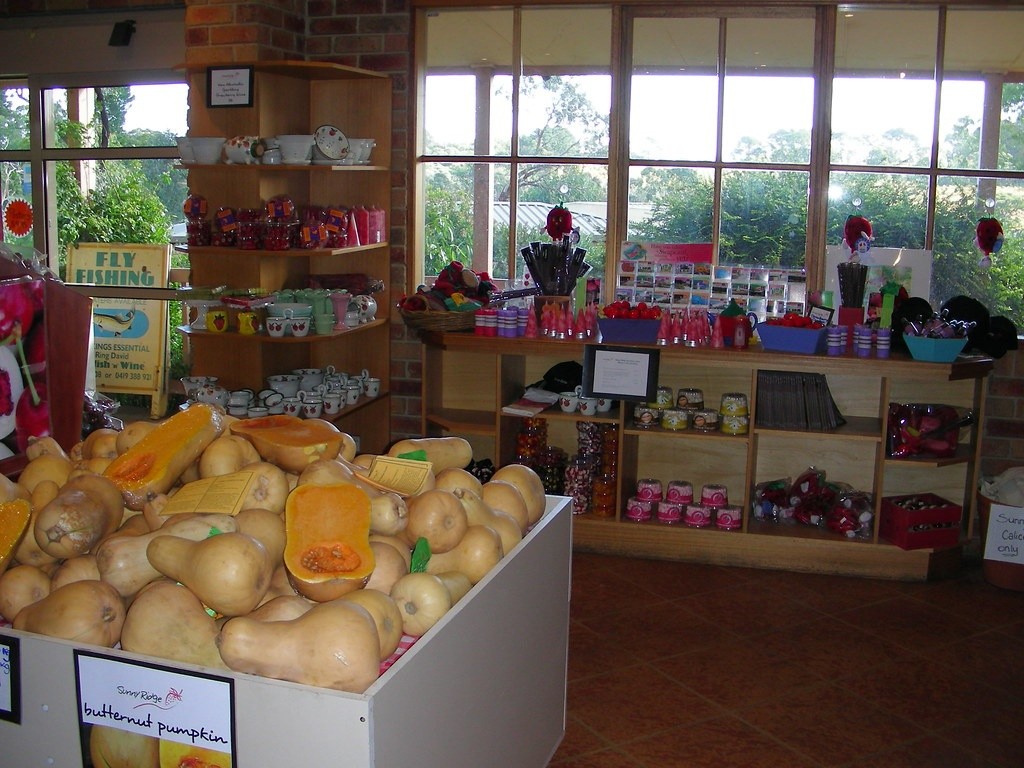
[626,386,749,530]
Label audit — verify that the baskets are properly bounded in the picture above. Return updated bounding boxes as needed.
[903,330,968,363]
[398,294,489,333]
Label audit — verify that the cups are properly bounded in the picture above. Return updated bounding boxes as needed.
[580,397,597,416]
[227,365,380,417]
[290,317,311,336]
[176,137,195,159]
[203,311,229,332]
[536,295,569,324]
[237,313,259,335]
[597,398,612,412]
[559,392,579,412]
[191,137,226,165]
[349,139,368,160]
[274,134,314,159]
[358,139,376,161]
[265,317,286,337]
[839,305,864,344]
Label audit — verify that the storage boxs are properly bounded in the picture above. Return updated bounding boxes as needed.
[0,492,575,768]
[879,494,963,552]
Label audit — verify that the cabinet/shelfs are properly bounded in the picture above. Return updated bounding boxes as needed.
[174,58,391,454]
[417,331,992,584]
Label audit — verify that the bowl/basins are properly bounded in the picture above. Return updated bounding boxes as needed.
[264,375,303,397]
[293,368,323,390]
[902,331,968,362]
[180,376,218,398]
[313,314,335,322]
[314,321,335,334]
[266,302,312,333]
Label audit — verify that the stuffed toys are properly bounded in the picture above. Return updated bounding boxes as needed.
[540,202,580,246]
[842,214,873,261]
[398,261,499,312]
[973,213,1004,268]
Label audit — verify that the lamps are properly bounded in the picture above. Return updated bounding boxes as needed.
[105,19,137,48]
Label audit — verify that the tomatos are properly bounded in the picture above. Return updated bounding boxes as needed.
[600,301,662,320]
[766,312,822,329]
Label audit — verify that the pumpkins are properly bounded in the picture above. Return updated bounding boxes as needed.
[89,723,233,768]
[0,401,548,694]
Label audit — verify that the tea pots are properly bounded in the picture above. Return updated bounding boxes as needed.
[707,300,757,347]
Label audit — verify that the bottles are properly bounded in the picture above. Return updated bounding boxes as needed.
[378,208,386,242]
[369,204,379,244]
[356,206,369,245]
[902,309,976,337]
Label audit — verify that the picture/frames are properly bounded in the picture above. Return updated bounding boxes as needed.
[807,304,835,328]
[825,244,932,324]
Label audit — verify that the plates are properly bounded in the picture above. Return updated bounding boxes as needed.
[354,161,363,164]
[315,125,350,159]
[284,159,311,165]
[363,161,371,164]
[312,160,354,165]
